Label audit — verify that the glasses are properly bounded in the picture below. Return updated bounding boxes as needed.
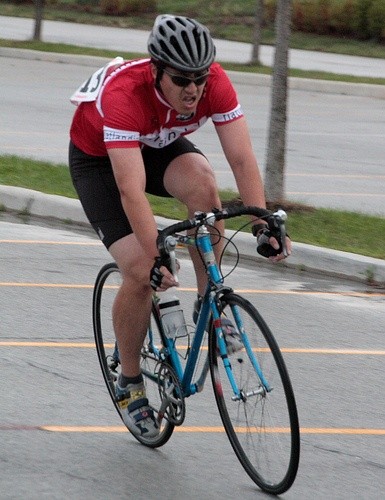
[163,69,210,87]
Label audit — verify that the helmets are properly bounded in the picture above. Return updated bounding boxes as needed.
[147,15,217,72]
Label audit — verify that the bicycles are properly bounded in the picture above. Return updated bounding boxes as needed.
[91,206,302,495]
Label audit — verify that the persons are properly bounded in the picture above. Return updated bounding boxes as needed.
[68,13,293,438]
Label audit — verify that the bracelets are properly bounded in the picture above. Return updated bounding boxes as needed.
[252,224,268,236]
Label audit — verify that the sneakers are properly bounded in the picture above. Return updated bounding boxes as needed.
[193,301,244,352]
[114,376,160,438]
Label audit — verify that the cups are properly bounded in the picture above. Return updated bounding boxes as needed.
[158,299,187,338]
